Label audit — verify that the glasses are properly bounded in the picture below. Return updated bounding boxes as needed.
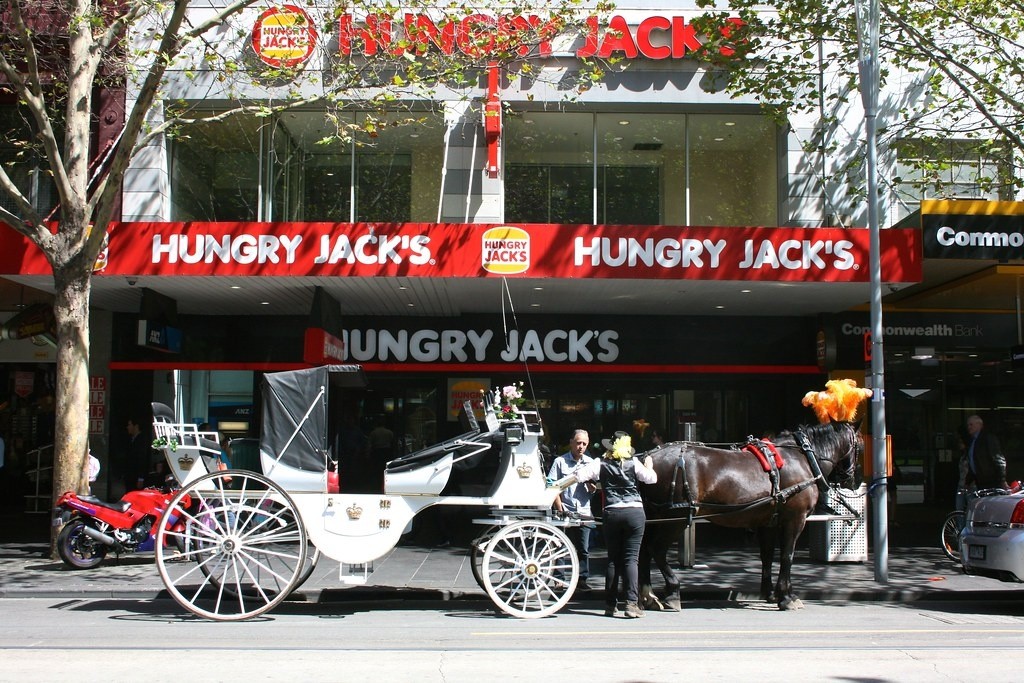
[652,435,657,438]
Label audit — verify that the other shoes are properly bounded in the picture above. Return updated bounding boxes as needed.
[624,603,645,618]
[554,580,564,592]
[577,580,599,599]
[604,604,618,617]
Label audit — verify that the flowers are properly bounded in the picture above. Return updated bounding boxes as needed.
[152,435,177,453]
[478,380,525,421]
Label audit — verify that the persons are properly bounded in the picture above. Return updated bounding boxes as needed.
[887,459,904,527]
[196,424,236,530]
[118,417,146,492]
[965,415,1011,492]
[144,462,171,490]
[651,429,668,446]
[368,418,393,467]
[952,437,978,556]
[601,431,657,617]
[548,429,599,592]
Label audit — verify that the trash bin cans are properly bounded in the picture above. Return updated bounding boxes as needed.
[809,480,869,564]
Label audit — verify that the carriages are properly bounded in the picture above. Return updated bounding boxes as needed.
[149,362,867,622]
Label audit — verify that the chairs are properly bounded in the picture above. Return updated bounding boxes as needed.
[151,402,222,490]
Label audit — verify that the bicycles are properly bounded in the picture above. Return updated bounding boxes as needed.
[940,488,1011,563]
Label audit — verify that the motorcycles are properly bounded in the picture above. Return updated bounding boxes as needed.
[49,474,204,571]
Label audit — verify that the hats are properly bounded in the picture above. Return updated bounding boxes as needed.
[601,431,636,456]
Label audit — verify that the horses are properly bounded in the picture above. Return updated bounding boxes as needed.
[633,413,866,610]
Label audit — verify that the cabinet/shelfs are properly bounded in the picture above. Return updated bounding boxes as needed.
[24,445,54,513]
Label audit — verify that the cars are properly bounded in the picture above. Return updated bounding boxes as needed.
[956,488,1024,585]
